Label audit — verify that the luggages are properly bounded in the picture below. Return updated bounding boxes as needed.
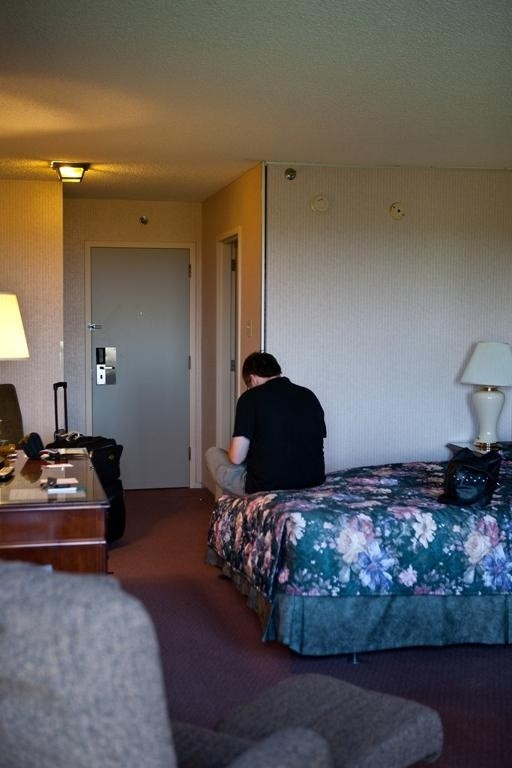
[45,382,125,543]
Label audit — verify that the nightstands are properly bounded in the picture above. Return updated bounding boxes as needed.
[446,442,511,459]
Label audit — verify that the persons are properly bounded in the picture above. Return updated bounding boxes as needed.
[205,352,326,499]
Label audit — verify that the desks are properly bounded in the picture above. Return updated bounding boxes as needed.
[1,447,111,575]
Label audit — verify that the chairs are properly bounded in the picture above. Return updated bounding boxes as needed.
[0,558,444,768]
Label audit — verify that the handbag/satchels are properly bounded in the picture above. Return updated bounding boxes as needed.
[437,448,502,507]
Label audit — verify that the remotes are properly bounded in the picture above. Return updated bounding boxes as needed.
[0,466,15,479]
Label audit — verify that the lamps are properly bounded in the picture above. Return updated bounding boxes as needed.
[462,341,511,445]
[0,294,30,359]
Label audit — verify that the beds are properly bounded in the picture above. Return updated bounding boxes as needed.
[206,459,512,655]
